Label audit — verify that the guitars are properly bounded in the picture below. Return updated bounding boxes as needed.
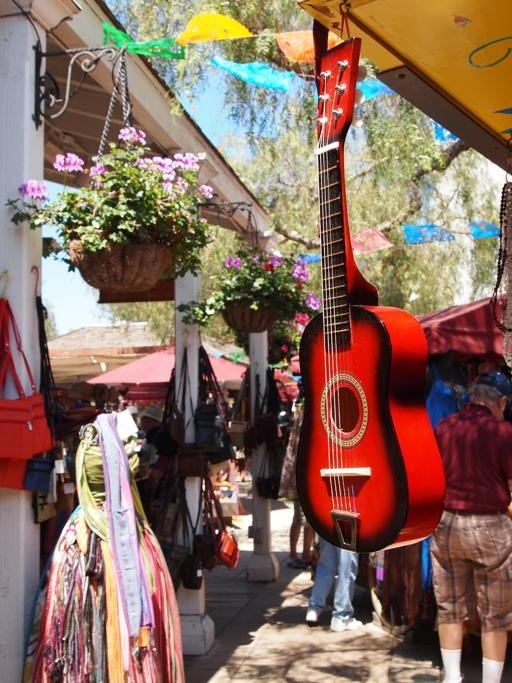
[296,36,446,554]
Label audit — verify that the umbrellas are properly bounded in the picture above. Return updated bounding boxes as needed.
[83,342,247,387]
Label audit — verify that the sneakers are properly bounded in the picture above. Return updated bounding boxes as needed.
[305,607,363,632]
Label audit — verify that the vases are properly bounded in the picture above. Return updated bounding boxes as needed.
[244,344,284,365]
[98,278,174,304]
[69,241,170,294]
[222,307,278,333]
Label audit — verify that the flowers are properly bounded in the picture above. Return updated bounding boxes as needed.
[173,235,320,328]
[233,323,301,372]
[4,124,218,278]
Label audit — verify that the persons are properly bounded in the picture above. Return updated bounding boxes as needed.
[429,368,511,683]
[303,537,365,632]
[276,381,320,570]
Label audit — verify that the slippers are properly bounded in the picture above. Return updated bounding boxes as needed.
[288,557,312,569]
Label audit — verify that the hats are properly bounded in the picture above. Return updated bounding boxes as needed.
[476,371,512,399]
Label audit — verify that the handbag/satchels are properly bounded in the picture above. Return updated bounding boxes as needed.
[0,391,74,522]
[159,529,239,592]
[146,405,290,512]
